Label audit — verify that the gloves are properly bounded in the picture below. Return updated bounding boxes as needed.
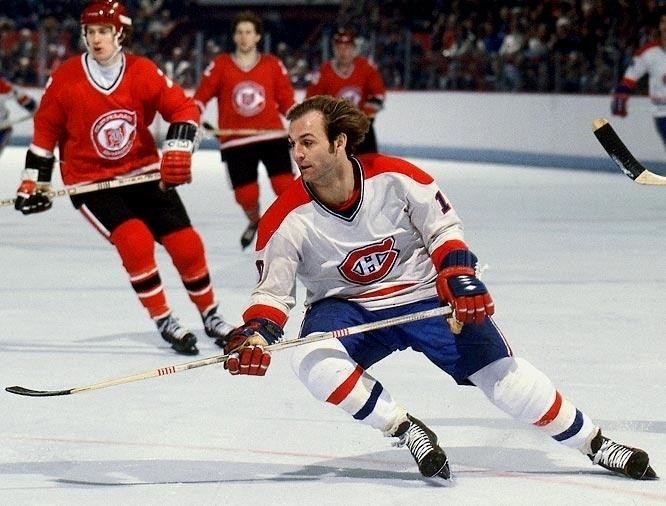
[435,249,496,326]
[159,121,198,187]
[611,85,632,118]
[13,148,57,216]
[223,304,290,376]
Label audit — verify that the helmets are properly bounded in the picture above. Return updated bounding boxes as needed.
[330,26,356,46]
[78,0,131,51]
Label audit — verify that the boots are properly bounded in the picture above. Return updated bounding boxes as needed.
[240,221,259,246]
[587,427,649,480]
[154,312,195,348]
[201,304,236,338]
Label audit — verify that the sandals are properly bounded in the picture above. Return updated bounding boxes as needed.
[386,411,448,479]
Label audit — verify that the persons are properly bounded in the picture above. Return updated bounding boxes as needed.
[302,32,386,156]
[0,77,36,154]
[0,0,666,96]
[191,12,301,249]
[610,14,666,152]
[16,0,239,350]
[223,96,649,480]
